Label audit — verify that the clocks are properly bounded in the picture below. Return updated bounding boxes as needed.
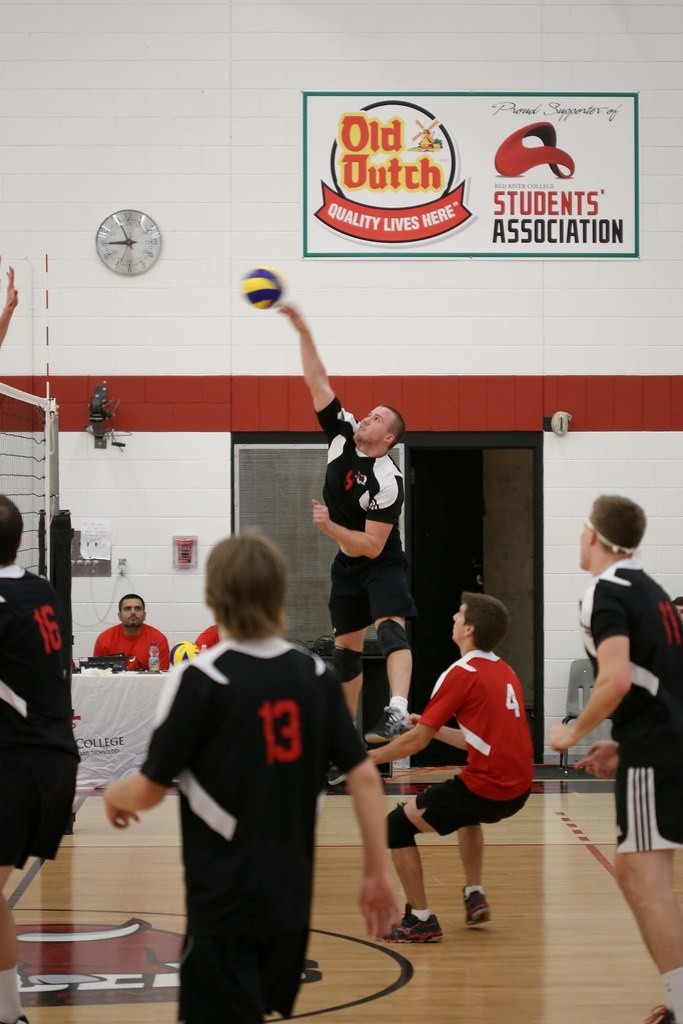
[95,210,161,277]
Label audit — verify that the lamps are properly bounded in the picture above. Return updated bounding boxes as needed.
[551,411,573,437]
[84,385,127,450]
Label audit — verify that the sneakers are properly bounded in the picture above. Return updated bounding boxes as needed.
[462,883,490,926]
[379,903,442,942]
[327,764,344,785]
[363,704,413,743]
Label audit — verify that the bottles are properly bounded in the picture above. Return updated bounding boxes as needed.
[199,644,207,655]
[149,643,159,673]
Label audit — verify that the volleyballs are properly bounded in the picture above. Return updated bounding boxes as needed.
[244,266,285,311]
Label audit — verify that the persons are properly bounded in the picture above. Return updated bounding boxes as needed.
[104,530,404,1024]
[546,494,683,1024]
[93,593,170,670]
[275,300,413,785]
[366,591,534,944]
[0,493,78,1024]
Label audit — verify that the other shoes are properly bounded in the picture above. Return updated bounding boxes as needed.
[643,1004,673,1024]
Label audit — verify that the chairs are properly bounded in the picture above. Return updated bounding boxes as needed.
[559,658,612,778]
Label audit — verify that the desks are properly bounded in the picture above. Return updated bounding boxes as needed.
[72,671,169,791]
